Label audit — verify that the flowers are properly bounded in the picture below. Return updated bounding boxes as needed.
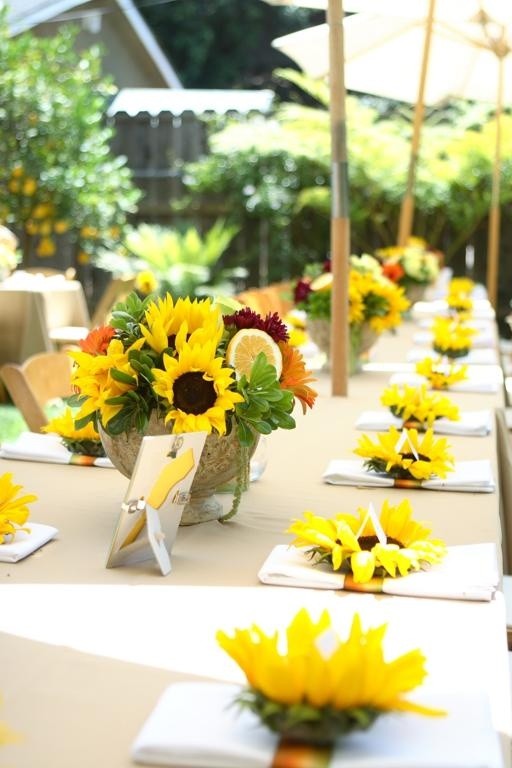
[71,278,317,433]
[41,407,107,457]
[376,233,436,285]
[215,609,447,745]
[0,471,40,544]
[281,253,412,335]
[287,500,444,585]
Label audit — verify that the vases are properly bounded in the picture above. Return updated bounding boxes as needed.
[99,405,265,520]
[306,313,380,373]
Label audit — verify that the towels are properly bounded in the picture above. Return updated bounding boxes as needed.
[257,543,504,602]
[0,522,58,563]
[326,278,502,492]
[0,431,116,468]
[128,682,492,768]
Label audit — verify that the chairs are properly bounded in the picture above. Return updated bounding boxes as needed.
[2,340,86,419]
